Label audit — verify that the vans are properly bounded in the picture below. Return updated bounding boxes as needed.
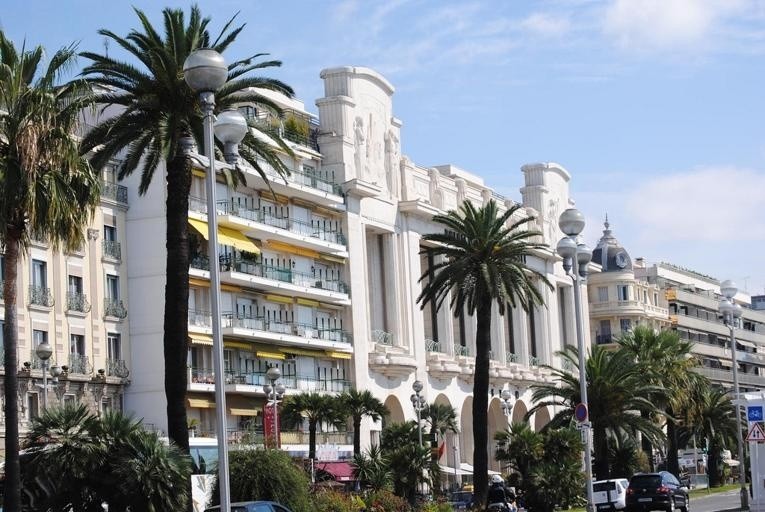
[591,477,629,510]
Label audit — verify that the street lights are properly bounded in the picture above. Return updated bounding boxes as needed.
[716,280,750,510]
[31,342,61,429]
[262,368,284,454]
[409,381,426,495]
[499,390,513,451]
[179,48,248,512]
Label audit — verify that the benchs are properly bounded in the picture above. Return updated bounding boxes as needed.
[446,491,473,510]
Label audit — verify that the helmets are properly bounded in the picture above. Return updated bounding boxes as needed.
[491,474,504,483]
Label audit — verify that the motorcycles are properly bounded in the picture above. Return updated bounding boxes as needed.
[487,495,520,512]
[556,209,597,512]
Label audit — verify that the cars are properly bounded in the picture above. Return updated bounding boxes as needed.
[446,491,474,512]
[206,499,290,512]
[625,471,690,512]
